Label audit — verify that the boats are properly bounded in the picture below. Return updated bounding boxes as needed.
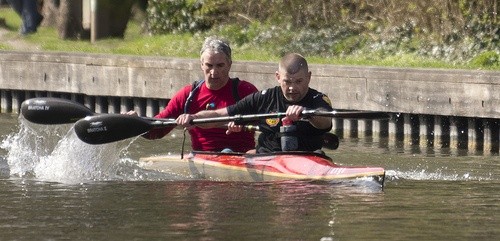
[139,147,386,183]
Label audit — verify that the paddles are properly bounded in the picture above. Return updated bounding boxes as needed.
[21,97,340,149]
[74,111,390,144]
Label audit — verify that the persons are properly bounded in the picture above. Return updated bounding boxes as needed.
[120,39,259,155]
[175,52,332,154]
